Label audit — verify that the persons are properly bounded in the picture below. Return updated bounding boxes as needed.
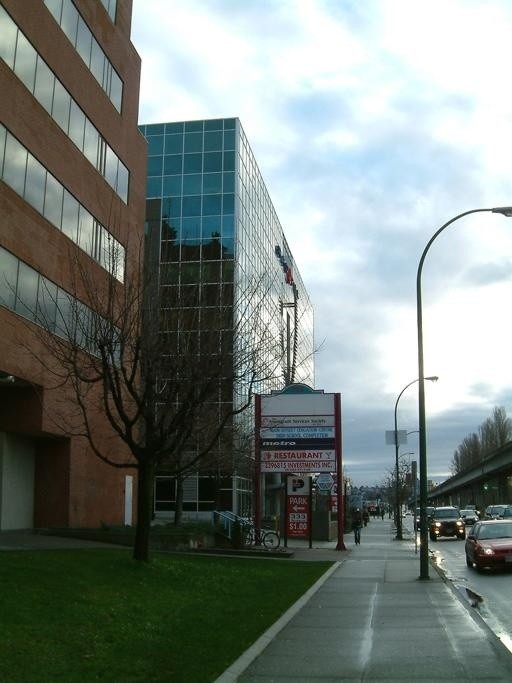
[351,507,363,545]
[363,504,392,526]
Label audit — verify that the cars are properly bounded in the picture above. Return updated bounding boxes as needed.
[414,505,512,574]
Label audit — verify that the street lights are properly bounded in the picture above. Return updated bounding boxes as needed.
[395,375,439,539]
[417,208,512,580]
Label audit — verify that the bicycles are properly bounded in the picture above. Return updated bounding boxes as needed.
[242,523,280,549]
[353,525,360,544]
